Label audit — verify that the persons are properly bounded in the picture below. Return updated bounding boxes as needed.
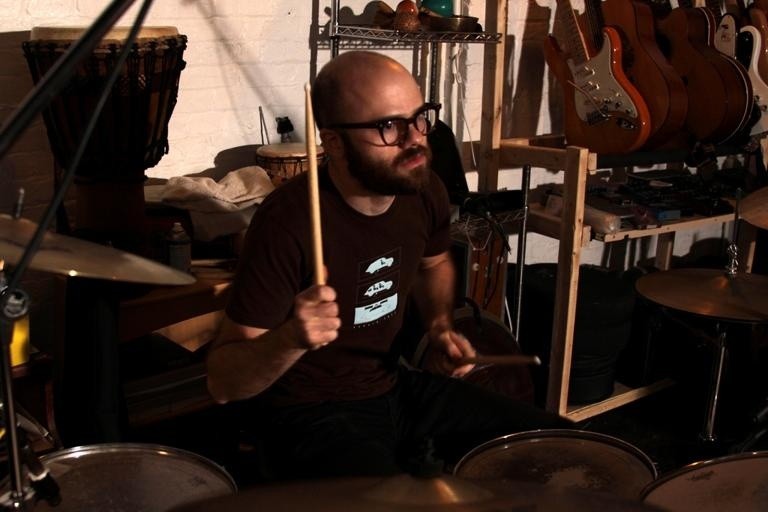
[203,51,581,476]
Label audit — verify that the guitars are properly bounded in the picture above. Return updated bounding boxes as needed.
[555,0,768,155]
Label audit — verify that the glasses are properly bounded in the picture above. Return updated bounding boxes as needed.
[339,102,445,147]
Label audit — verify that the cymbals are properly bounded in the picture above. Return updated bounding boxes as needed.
[738,187,768,230]
[635,267,768,320]
[0,218,197,285]
[169,473,657,511]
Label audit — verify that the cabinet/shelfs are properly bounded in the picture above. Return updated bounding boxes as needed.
[478,0,758,427]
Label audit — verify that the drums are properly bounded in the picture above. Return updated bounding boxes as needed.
[635,448,768,512]
[25,26,187,253]
[256,142,324,187]
[451,428,656,511]
[0,444,237,511]
